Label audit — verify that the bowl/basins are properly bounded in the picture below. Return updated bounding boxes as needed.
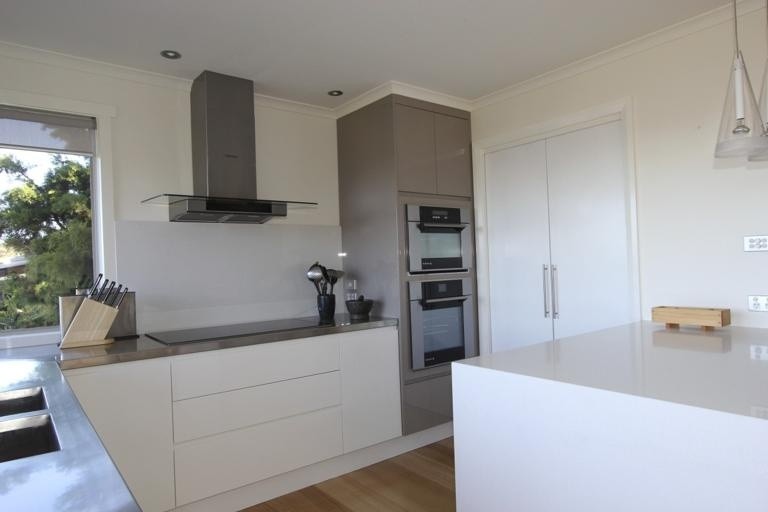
[345,298,373,320]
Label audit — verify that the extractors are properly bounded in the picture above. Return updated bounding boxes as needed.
[139,70,318,225]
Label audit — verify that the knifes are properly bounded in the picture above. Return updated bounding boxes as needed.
[87,273,129,309]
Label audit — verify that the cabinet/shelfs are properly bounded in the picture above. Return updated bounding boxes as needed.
[61,325,401,512]
[484,119,635,358]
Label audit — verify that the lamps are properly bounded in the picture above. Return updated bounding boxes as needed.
[712,0,768,159]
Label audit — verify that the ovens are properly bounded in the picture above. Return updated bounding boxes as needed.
[404,202,476,371]
